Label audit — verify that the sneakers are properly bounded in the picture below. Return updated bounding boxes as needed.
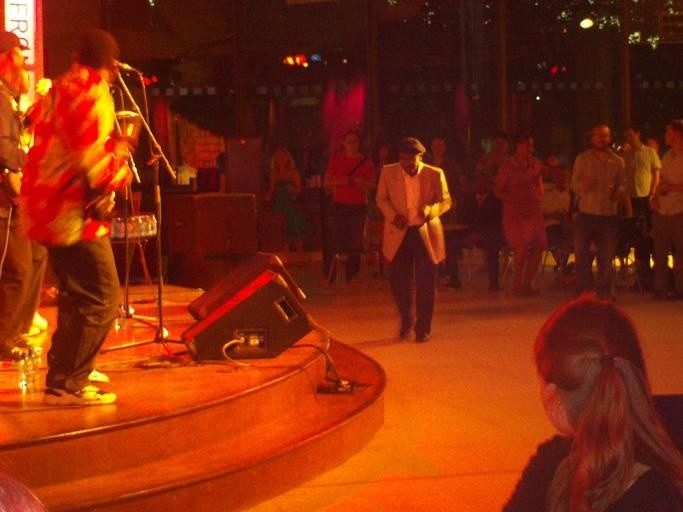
[1,311,48,361]
[43,369,117,406]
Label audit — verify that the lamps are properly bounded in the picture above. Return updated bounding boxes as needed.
[572,8,593,29]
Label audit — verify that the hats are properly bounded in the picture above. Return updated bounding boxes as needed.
[396,138,425,152]
[1,32,31,51]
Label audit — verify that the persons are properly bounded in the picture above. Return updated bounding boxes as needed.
[502,292,683,512]
[20,26,141,405]
[1,30,45,359]
[266,150,309,267]
[428,119,682,300]
[325,131,377,282]
[377,135,453,343]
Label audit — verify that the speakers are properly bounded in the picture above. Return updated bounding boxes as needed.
[227,136,267,209]
[180,270,315,361]
[165,190,257,286]
[186,250,306,317]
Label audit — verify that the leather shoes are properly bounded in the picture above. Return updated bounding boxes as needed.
[399,325,431,342]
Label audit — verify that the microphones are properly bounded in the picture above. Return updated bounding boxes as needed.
[115,62,134,74]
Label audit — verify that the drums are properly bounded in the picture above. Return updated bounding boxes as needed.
[112,212,157,241]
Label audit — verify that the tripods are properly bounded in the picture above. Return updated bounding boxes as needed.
[97,74,186,358]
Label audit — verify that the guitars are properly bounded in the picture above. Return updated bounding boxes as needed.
[81,109,143,222]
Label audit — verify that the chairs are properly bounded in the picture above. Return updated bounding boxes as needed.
[325,218,646,301]
